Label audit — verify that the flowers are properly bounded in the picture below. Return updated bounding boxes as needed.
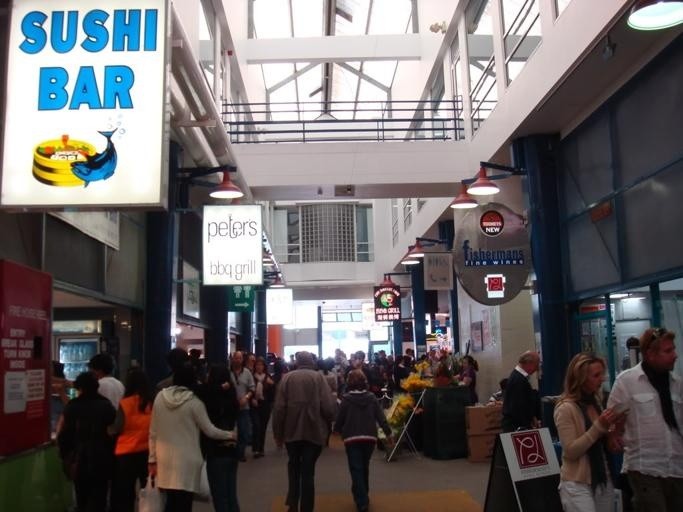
[383,345,454,427]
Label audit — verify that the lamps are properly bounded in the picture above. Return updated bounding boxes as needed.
[264,270,285,288]
[401,238,450,266]
[450,162,527,210]
[174,164,244,205]
[626,0,683,32]
[382,274,418,288]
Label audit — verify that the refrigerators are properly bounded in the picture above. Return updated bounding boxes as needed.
[49,319,105,403]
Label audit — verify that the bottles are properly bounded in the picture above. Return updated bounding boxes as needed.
[57,341,96,399]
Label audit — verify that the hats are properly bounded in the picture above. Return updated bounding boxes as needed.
[294,351,318,370]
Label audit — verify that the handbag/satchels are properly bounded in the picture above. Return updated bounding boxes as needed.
[137,471,166,512]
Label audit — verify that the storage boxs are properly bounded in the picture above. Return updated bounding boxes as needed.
[465,404,504,460]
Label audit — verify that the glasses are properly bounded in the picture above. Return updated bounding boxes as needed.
[645,327,666,351]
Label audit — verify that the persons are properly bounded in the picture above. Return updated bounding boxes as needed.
[552,351,627,510]
[452,354,480,403]
[486,376,509,403]
[618,334,643,371]
[196,363,245,511]
[500,349,545,435]
[331,367,393,511]
[214,337,449,463]
[606,323,682,510]
[271,351,336,511]
[144,361,240,511]
[43,336,201,512]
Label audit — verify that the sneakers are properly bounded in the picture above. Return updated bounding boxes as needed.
[254,451,264,458]
[239,457,246,462]
[288,506,298,512]
[359,503,368,512]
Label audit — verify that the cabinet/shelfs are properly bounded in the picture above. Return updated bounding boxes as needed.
[422,387,473,460]
